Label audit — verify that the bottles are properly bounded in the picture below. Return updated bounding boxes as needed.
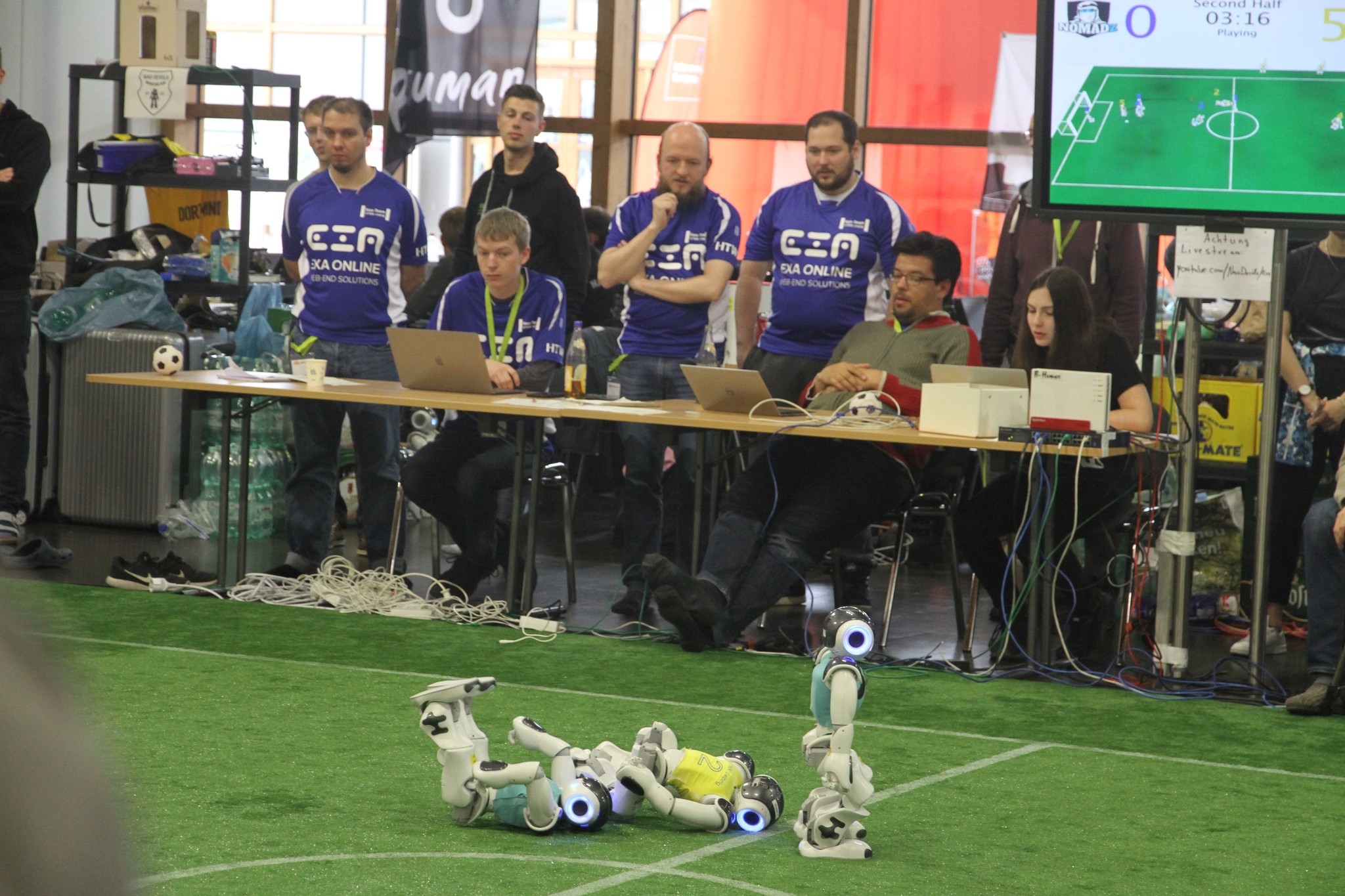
[158,508,194,537]
[253,357,294,448]
[270,445,295,534]
[248,444,274,539]
[197,443,239,538]
[204,355,253,447]
[564,320,587,399]
[83,286,118,318]
[50,303,83,331]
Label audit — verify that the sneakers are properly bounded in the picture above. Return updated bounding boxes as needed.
[105,551,217,592]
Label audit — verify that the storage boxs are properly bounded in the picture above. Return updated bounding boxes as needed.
[92,141,166,173]
[919,383,1029,438]
[210,228,240,284]
[46,237,96,262]
[1153,376,1264,463]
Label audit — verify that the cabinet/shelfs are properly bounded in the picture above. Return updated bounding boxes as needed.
[62,65,301,532]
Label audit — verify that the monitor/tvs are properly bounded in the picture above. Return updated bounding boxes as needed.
[1034,0,1345,228]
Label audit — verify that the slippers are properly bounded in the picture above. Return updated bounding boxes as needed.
[3,536,72,568]
[0,511,19,543]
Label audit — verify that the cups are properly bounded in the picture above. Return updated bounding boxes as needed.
[306,358,328,387]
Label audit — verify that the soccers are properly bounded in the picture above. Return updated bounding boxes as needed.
[849,393,883,419]
[150,343,184,376]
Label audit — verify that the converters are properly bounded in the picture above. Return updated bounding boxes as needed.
[392,605,434,620]
[520,616,566,634]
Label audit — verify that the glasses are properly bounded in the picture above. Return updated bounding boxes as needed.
[889,268,939,285]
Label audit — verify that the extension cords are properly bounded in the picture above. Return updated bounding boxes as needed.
[311,581,341,607]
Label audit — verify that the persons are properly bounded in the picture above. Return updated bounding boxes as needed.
[264,97,428,579]
[279,83,625,345]
[642,230,983,652]
[599,120,742,617]
[733,111,914,612]
[0,49,50,545]
[401,207,566,612]
[954,175,1153,668]
[1227,230,1345,714]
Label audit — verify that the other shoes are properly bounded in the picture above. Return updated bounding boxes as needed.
[390,571,412,592]
[496,519,537,601]
[263,564,301,579]
[1285,678,1345,715]
[430,552,499,599]
[1230,625,1288,656]
[1055,592,1116,659]
[990,608,1029,659]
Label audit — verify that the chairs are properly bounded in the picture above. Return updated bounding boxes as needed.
[355,326,984,615]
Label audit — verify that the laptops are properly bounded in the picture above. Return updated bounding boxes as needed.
[680,364,814,416]
[386,327,524,395]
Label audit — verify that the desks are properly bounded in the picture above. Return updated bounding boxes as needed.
[86,371,1181,687]
